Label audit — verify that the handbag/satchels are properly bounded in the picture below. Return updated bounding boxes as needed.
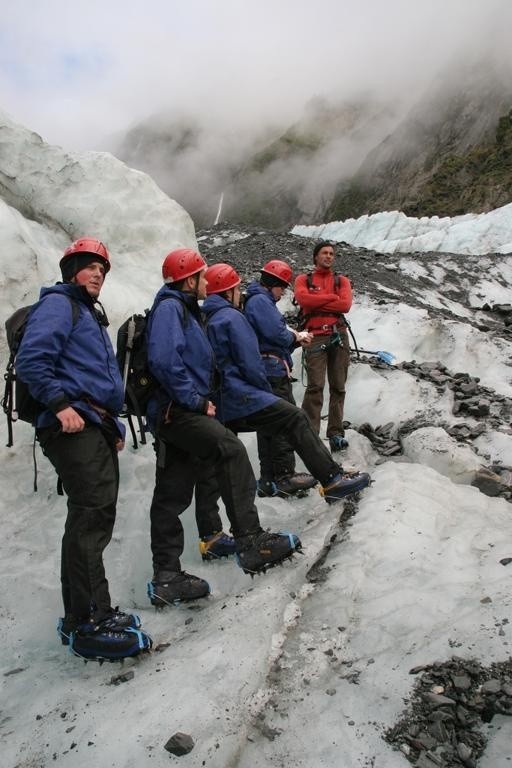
[283,310,305,332]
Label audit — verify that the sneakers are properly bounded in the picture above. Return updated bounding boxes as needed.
[58,607,141,646]
[274,470,317,498]
[200,531,238,561]
[68,623,154,667]
[146,569,210,613]
[323,471,370,505]
[257,478,275,498]
[237,530,302,580]
[330,436,349,453]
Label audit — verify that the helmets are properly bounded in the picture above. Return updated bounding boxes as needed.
[261,260,293,287]
[59,239,111,272]
[204,263,241,294]
[162,248,208,284]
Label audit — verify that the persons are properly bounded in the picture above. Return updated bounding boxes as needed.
[293,244,352,450]
[244,260,319,496]
[15,238,152,662]
[195,264,370,562]
[143,248,299,606]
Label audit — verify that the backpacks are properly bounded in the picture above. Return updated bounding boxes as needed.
[2,291,79,423]
[115,292,189,449]
